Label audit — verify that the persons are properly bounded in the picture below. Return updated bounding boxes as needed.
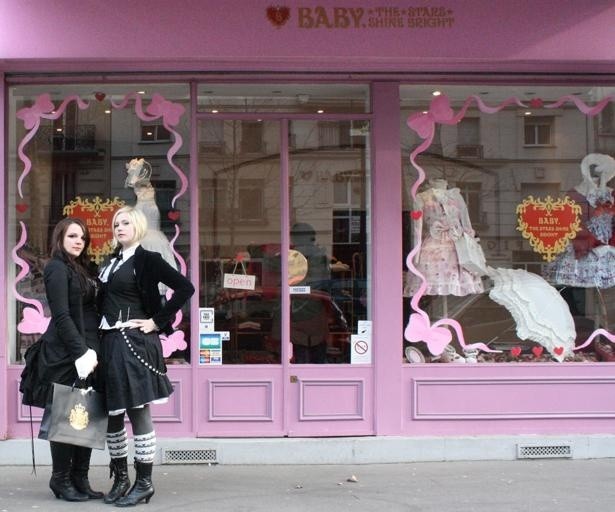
[545,153,615,289]
[265,222,331,364]
[97,206,195,506]
[403,177,486,297]
[19,218,103,500]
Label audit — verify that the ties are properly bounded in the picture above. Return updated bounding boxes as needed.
[108,253,122,280]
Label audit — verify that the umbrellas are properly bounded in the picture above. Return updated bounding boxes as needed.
[477,265,577,363]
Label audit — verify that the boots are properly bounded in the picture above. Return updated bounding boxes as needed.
[72,444,104,499]
[103,455,130,504]
[48,441,90,502]
[114,459,155,507]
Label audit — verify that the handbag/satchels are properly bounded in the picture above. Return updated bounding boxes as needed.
[38,378,110,451]
